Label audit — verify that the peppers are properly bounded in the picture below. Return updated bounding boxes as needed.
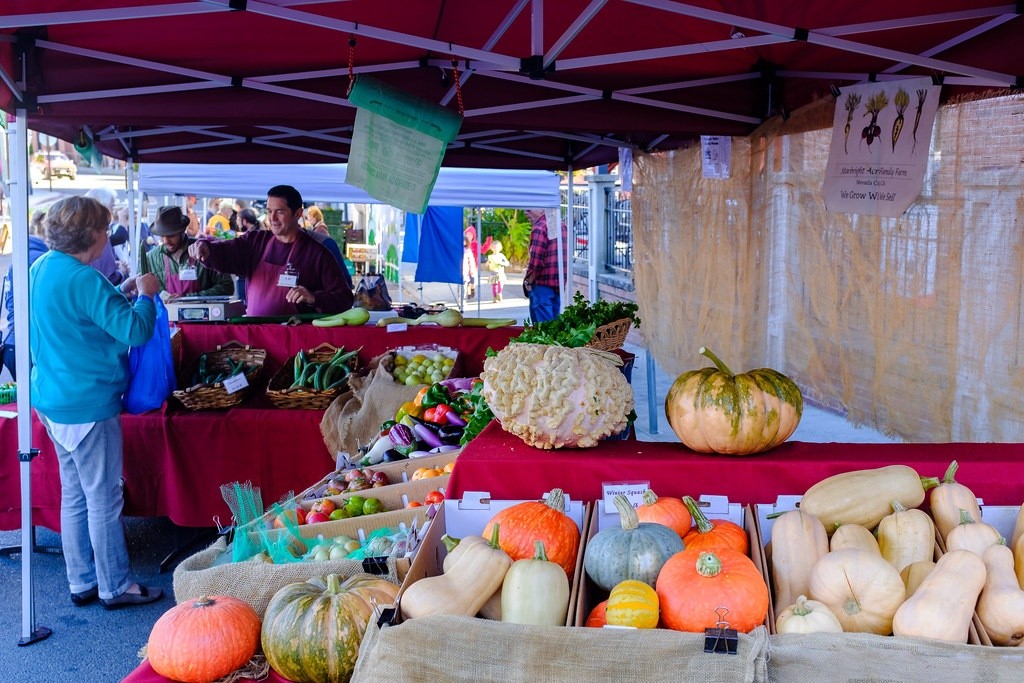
[380,377,483,430]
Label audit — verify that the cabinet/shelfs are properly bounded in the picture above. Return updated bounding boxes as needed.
[120,419,1024,683]
[0,320,636,574]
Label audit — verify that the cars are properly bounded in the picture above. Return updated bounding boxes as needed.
[573,198,634,269]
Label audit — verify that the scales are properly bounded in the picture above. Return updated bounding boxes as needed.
[164,295,242,324]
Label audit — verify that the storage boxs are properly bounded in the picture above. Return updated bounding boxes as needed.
[172,444,1024,683]
[321,209,378,262]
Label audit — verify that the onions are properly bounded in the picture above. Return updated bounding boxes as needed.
[252,536,361,564]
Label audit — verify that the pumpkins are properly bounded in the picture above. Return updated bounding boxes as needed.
[478,341,633,449]
[400,460,1024,647]
[665,346,805,456]
[261,573,401,683]
[147,595,262,683]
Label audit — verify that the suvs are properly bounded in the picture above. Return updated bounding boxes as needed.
[32,150,76,180]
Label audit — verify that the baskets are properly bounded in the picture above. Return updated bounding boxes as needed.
[585,318,632,352]
[172,340,267,412]
[266,342,359,410]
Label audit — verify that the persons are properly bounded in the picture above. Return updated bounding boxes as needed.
[0,189,353,381]
[457,207,568,328]
[28,196,165,609]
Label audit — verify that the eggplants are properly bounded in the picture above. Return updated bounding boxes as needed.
[361,412,469,466]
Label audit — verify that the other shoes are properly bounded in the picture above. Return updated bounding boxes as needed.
[493,296,497,302]
[99,583,164,609]
[497,294,502,301]
[70,585,98,605]
[468,295,471,299]
[471,289,475,296]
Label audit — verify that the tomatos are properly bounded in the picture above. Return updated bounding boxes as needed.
[392,354,455,386]
[273,463,456,529]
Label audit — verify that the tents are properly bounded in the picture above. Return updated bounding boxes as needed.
[32,163,570,317]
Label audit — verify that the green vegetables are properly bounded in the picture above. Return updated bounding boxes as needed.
[460,290,641,447]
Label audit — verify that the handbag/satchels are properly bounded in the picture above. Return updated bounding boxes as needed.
[120,292,177,415]
[352,273,393,311]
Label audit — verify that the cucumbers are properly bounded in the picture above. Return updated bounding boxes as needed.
[192,355,259,388]
[289,346,363,391]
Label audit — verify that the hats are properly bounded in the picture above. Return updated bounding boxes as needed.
[148,206,190,237]
[219,200,238,213]
